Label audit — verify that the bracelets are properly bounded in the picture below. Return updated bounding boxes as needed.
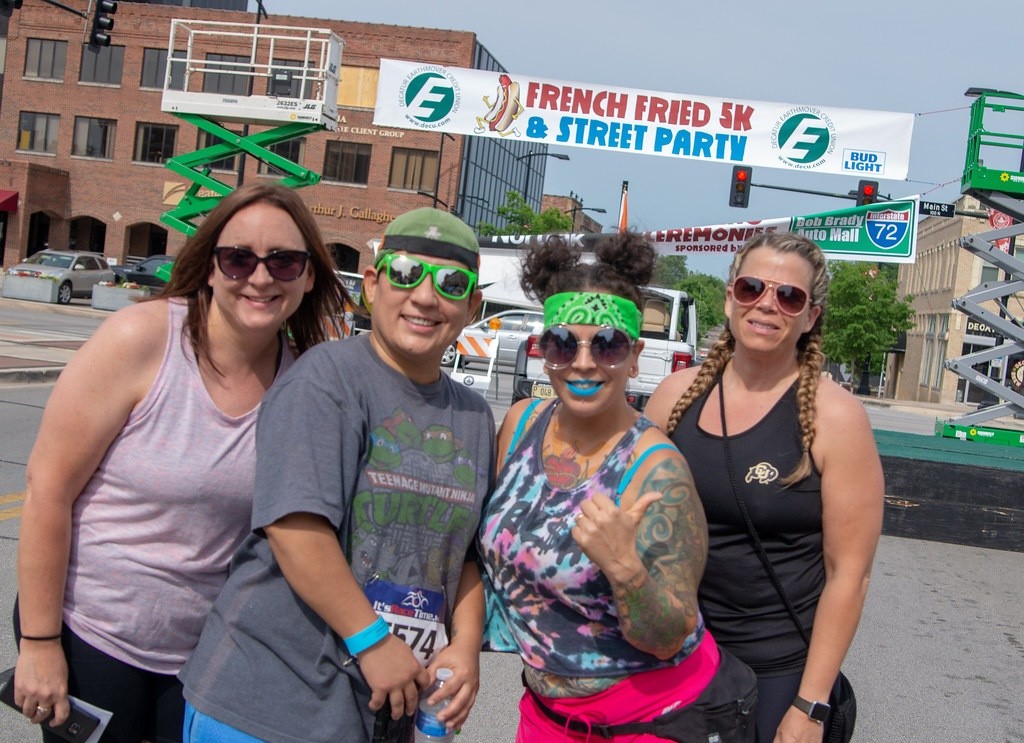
[20,632,63,641]
[343,616,390,656]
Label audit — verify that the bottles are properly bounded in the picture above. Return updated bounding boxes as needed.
[412,668,457,742]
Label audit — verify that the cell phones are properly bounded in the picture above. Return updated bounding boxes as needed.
[0,674,101,743]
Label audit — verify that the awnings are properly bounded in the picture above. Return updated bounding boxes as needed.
[0,189,19,215]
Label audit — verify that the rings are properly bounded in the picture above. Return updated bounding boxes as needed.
[575,514,584,521]
[36,706,48,712]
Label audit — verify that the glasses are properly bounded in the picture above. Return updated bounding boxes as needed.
[536,326,638,369]
[377,253,478,300]
[728,275,812,317]
[212,246,314,281]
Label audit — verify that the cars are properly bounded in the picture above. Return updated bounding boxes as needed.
[439,311,545,368]
[13,248,116,305]
[331,270,366,307]
[108,254,178,295]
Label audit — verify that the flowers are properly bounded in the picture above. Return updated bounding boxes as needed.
[4,269,59,282]
[98,281,148,289]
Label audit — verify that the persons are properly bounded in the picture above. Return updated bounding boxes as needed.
[11,168,369,743]
[644,233,888,743]
[466,232,720,743]
[177,204,501,743]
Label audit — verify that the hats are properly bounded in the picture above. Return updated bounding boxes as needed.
[361,207,479,315]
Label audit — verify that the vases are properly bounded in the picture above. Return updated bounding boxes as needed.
[2,275,59,303]
[91,284,150,311]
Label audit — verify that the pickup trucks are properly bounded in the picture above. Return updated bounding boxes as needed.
[511,284,698,408]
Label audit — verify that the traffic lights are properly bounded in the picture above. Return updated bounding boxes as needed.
[86,0,118,55]
[0,0,23,17]
[728,165,752,209]
[854,180,878,208]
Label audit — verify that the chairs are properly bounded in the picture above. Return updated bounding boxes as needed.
[639,299,670,333]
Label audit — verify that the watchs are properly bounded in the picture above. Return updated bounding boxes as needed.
[792,694,832,723]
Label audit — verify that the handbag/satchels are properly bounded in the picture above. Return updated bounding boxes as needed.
[646,645,758,742]
[826,671,856,743]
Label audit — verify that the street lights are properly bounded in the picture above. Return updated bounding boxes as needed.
[516,150,571,204]
[962,87,1024,413]
[564,206,607,236]
